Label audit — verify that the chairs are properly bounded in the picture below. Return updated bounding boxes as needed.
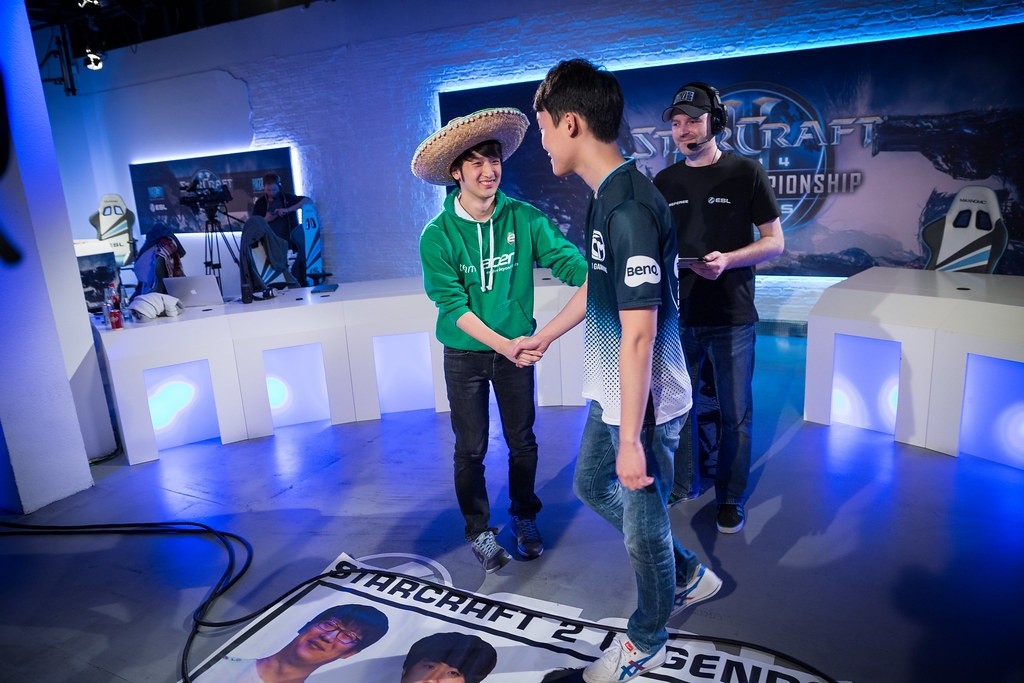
[921,186,1008,273]
[241,205,333,291]
[88,195,137,266]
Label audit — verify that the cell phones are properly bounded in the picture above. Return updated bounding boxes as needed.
[678,257,705,268]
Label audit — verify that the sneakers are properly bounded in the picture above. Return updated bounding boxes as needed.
[666,492,699,509]
[509,516,543,557]
[667,564,723,620]
[715,503,744,535]
[583,629,666,683]
[470,531,512,575]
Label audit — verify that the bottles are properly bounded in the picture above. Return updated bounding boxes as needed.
[101,283,133,330]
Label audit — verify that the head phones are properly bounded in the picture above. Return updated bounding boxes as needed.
[680,81,728,136]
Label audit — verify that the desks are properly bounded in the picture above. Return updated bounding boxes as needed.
[802,266,1023,457]
[89,268,588,466]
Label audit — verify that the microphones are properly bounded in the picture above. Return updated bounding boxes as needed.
[686,127,721,149]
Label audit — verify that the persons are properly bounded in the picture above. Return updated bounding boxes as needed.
[252,172,314,282]
[514,58,693,683]
[191,605,389,683]
[654,81,786,534]
[411,107,588,574]
[400,633,497,683]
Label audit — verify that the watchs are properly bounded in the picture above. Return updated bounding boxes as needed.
[285,209,288,213]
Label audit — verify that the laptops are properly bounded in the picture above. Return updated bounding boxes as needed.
[163,275,234,308]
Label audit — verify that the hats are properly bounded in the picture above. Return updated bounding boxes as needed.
[663,85,719,122]
[410,107,530,186]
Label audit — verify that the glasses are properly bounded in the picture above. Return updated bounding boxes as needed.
[318,619,362,645]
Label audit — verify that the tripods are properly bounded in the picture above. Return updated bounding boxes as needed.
[202,206,247,297]
